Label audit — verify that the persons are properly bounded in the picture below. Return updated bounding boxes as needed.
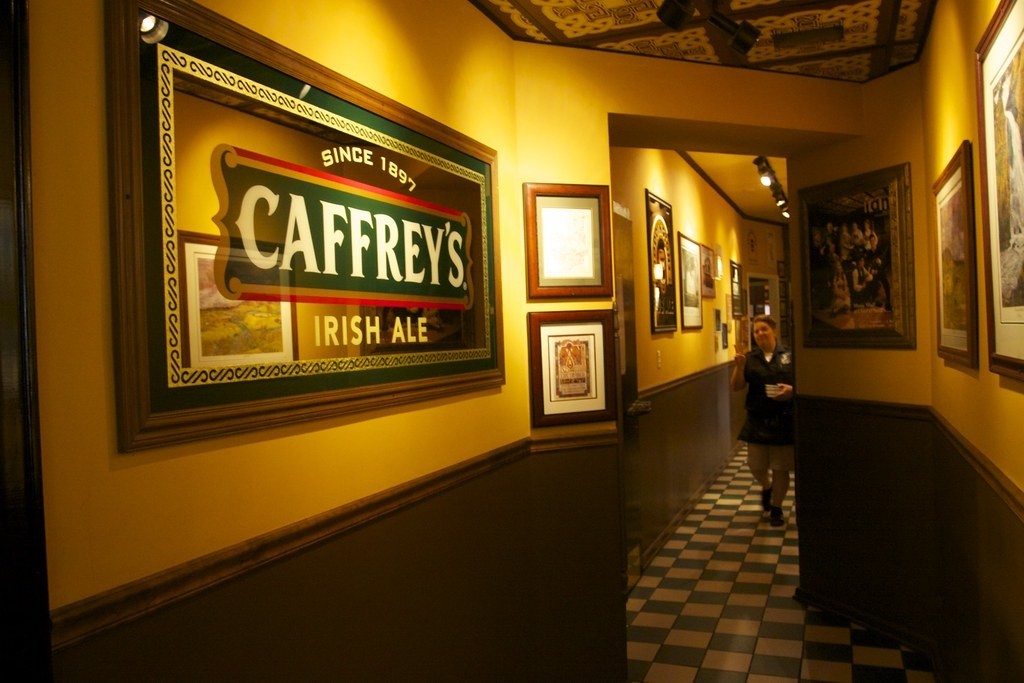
[730,313,795,528]
[813,214,892,318]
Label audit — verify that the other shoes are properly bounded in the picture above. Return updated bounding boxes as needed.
[770,506,784,527]
[762,487,773,512]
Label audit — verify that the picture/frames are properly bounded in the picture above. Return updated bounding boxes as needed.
[974,0,1024,383]
[644,187,678,334]
[104,0,507,454]
[528,309,618,428]
[701,243,717,298]
[797,163,917,348]
[522,182,613,298]
[933,140,978,370]
[678,231,704,330]
